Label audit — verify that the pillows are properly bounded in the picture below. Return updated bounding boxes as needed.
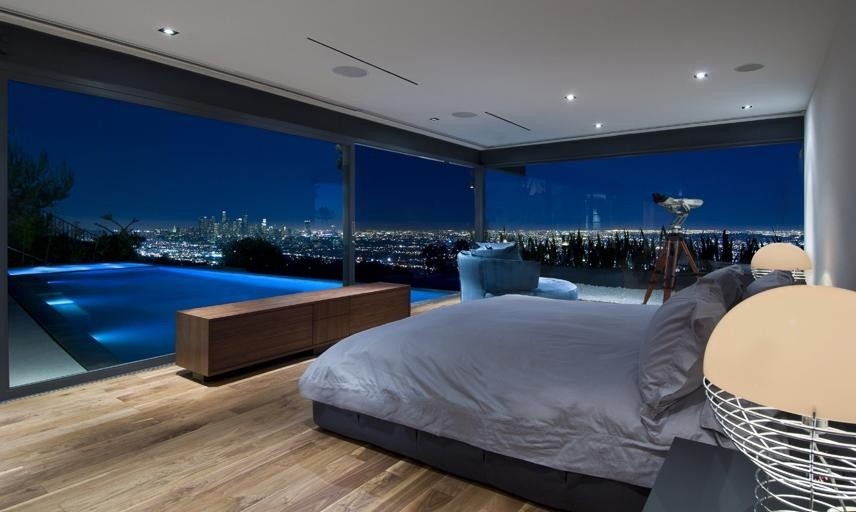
[638,264,797,411]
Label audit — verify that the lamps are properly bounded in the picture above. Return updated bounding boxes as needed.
[751,243,812,283]
[702,283,856,512]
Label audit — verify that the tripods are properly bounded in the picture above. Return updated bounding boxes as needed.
[642,231,701,304]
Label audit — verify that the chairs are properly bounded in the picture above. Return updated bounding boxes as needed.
[457,241,577,306]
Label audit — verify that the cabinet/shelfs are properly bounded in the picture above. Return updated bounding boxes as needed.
[175,281,412,384]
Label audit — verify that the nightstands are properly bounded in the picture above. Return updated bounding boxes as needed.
[640,436,850,512]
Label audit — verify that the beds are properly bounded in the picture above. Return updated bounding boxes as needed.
[312,293,778,511]
